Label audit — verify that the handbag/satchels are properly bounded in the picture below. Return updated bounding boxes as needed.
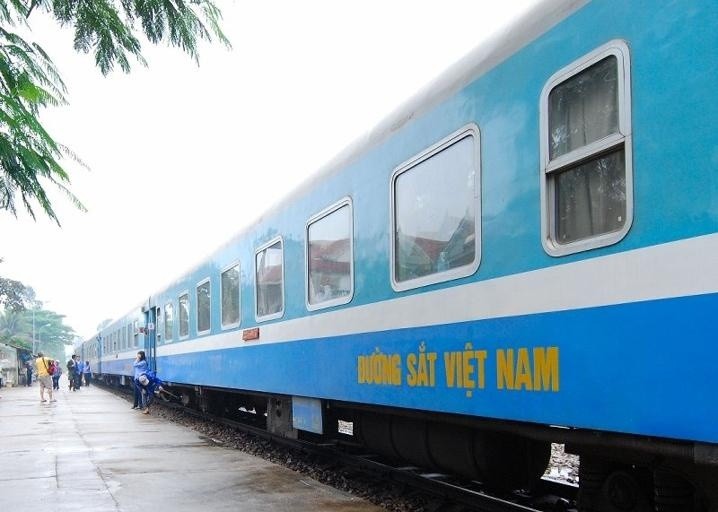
[46,364,58,376]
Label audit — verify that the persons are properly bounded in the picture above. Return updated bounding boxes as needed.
[35,351,57,403]
[131,350,150,410]
[136,372,171,415]
[24,351,93,392]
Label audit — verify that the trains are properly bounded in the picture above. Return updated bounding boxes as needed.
[66,0,718,512]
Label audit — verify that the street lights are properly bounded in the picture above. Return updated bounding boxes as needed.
[39,323,51,351]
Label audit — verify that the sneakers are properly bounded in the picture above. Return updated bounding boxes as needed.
[132,404,149,415]
[40,398,57,402]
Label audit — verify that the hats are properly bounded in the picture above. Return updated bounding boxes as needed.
[138,376,150,386]
